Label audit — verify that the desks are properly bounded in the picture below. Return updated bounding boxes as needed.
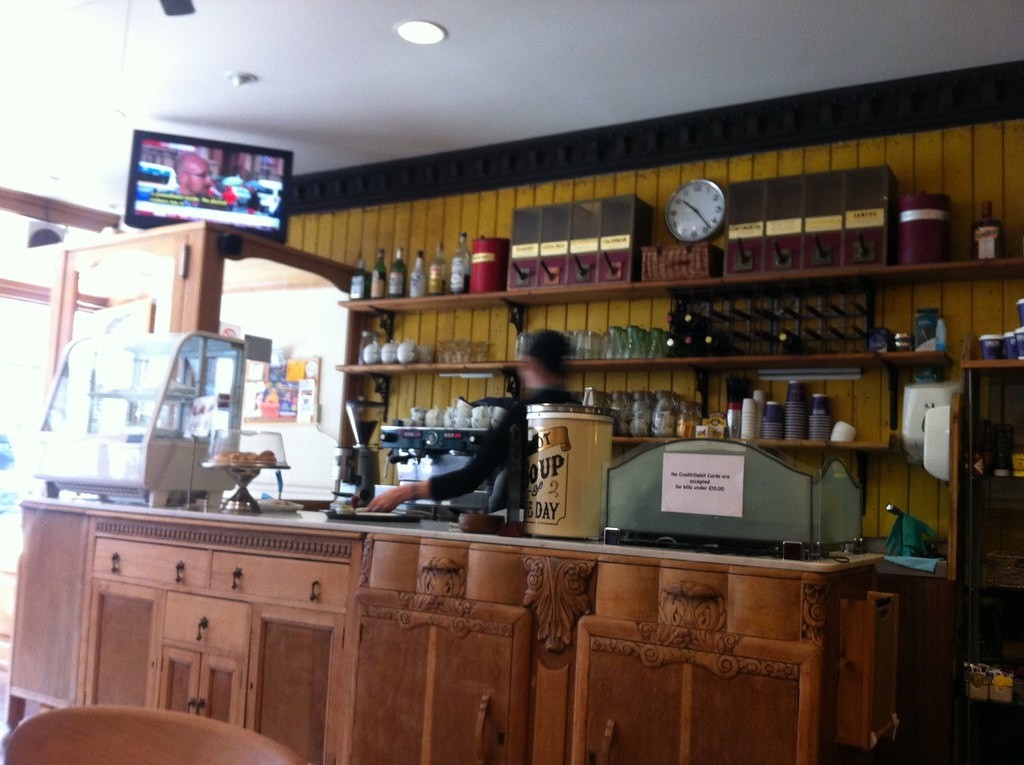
[7,496,254,728]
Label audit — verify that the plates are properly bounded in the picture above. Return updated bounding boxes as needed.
[257,502,304,510]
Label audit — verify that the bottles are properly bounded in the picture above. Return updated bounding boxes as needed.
[973,200,1002,259]
[779,333,810,354]
[684,314,707,332]
[349,260,370,299]
[705,336,722,356]
[666,316,686,333]
[666,339,686,357]
[370,249,387,298]
[973,438,984,475]
[410,251,426,297]
[685,336,703,356]
[449,233,470,294]
[388,247,407,297]
[936,320,946,350]
[595,390,703,438]
[983,420,993,475]
[428,241,447,295]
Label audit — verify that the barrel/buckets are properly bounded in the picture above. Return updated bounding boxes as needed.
[526,403,615,538]
[471,238,511,294]
[900,194,950,264]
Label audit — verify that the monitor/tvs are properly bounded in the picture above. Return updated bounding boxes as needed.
[123,129,294,243]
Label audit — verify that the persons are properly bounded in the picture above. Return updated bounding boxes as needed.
[365,329,583,514]
[171,151,259,215]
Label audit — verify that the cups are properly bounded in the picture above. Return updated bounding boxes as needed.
[727,379,831,440]
[441,340,486,363]
[560,326,667,359]
[980,334,1003,359]
[1005,332,1016,359]
[1017,300,1024,325]
[404,396,504,429]
[1014,328,1024,359]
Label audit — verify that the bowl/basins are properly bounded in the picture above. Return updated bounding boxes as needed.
[459,513,504,534]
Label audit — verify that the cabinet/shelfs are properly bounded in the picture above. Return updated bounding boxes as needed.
[347,533,889,765]
[76,509,364,765]
[339,261,1024,454]
[961,360,1023,763]
[32,328,245,432]
